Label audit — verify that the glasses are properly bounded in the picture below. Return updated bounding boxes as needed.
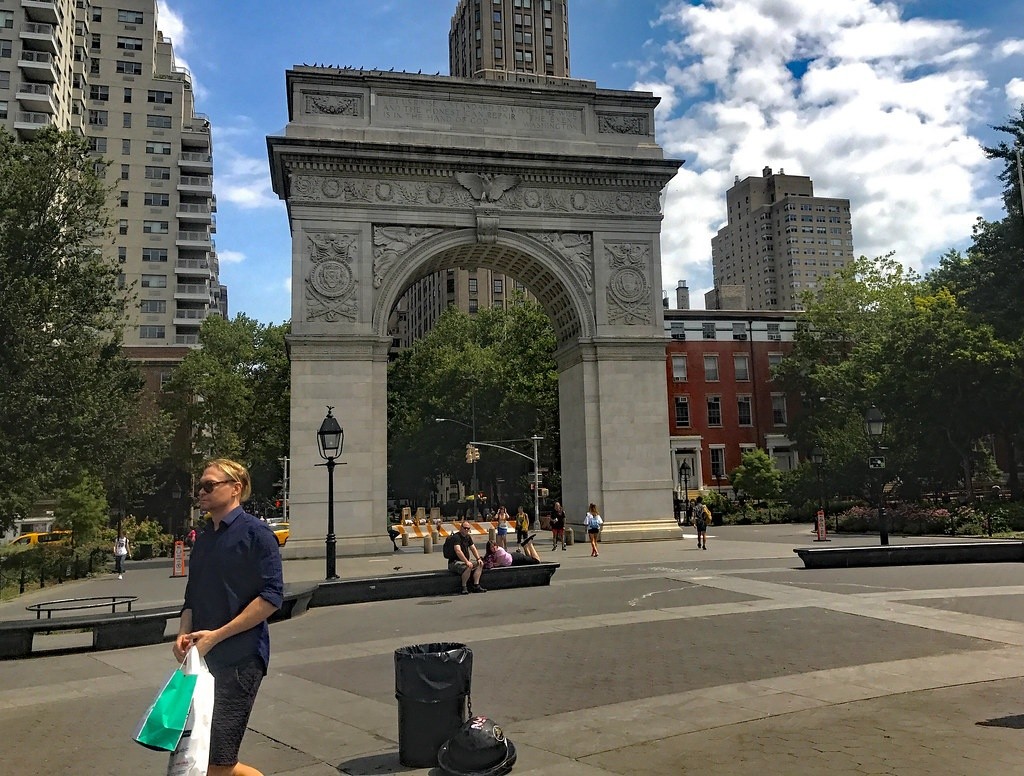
[462,526,470,530]
[198,480,238,493]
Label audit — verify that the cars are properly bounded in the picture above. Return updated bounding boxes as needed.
[268,523,290,547]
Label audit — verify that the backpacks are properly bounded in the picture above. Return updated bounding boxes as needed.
[442,532,465,559]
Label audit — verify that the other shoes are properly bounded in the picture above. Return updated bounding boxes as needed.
[594,553,598,557]
[562,548,567,550]
[552,547,556,551]
[702,546,707,550]
[461,586,469,594]
[590,553,594,556]
[118,575,122,579]
[472,586,487,592]
[697,543,702,549]
[521,533,537,547]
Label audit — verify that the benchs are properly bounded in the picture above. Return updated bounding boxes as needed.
[0,581,317,661]
[793,541,1024,568]
[310,562,560,609]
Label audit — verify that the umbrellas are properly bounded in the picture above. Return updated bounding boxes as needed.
[464,495,481,505]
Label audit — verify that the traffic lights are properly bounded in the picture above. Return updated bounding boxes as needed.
[465,444,472,464]
[472,447,481,460]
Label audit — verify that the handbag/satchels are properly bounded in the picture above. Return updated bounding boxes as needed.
[702,506,712,524]
[168,646,216,776]
[131,651,199,752]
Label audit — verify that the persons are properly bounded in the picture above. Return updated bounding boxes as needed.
[550,502,567,551]
[413,505,552,530]
[673,496,712,551]
[114,528,131,580]
[448,522,487,594]
[483,534,541,567]
[185,526,195,557]
[515,506,529,552]
[173,460,284,776]
[584,503,603,557]
[495,506,509,551]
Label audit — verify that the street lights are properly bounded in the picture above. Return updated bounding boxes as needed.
[315,405,347,580]
[435,418,478,522]
[715,471,722,494]
[810,444,824,533]
[860,401,891,545]
[678,458,692,526]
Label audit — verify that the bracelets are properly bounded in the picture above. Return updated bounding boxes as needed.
[476,558,481,560]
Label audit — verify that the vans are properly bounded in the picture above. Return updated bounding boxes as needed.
[6,530,73,548]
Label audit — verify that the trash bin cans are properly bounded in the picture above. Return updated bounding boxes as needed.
[394,642,517,776]
[713,512,722,525]
